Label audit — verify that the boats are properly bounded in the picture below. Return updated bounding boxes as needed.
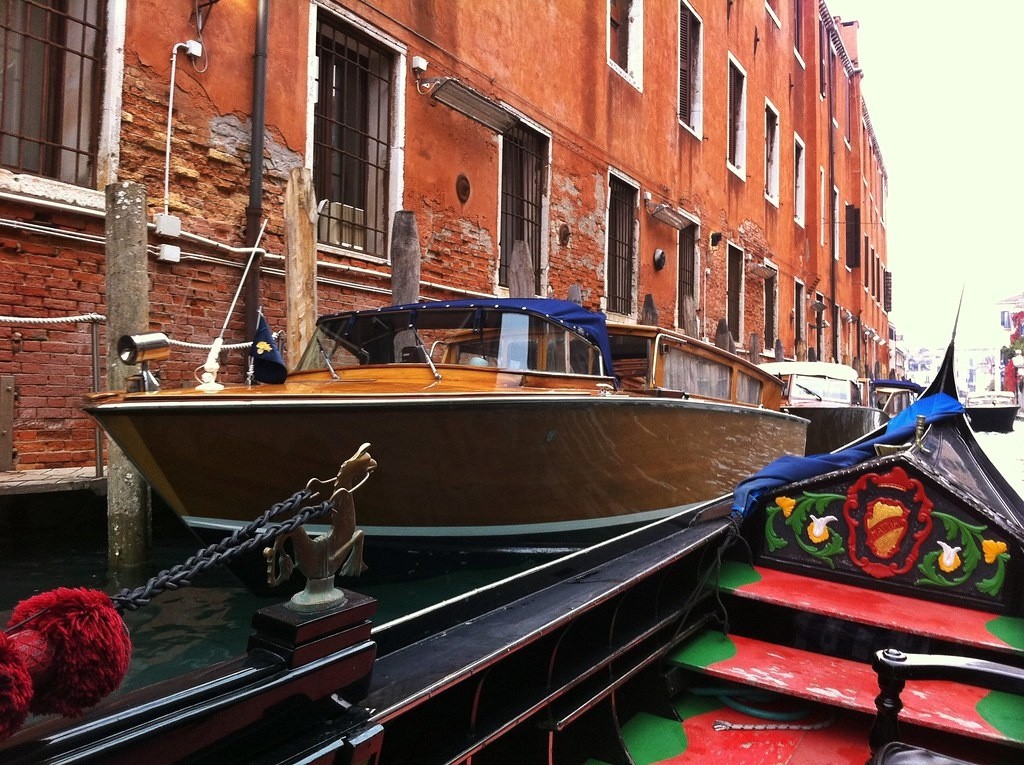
[756,360,885,456]
[83,299,810,585]
[2,292,1024,765]
[963,391,1021,434]
